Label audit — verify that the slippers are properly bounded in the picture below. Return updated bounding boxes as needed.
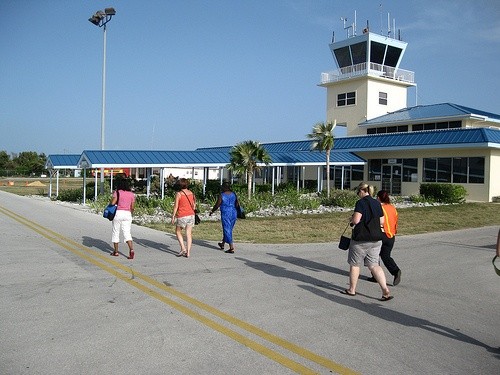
[379,296,393,300]
[340,288,356,296]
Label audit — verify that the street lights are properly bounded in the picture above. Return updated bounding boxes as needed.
[87,6,116,148]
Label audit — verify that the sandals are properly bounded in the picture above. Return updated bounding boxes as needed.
[225,248,234,253]
[128,249,134,259]
[218,243,224,249]
[110,250,120,256]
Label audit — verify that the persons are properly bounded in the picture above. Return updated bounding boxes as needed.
[171,178,196,258]
[208,182,240,254]
[108,178,135,259]
[340,181,394,301]
[367,190,402,287]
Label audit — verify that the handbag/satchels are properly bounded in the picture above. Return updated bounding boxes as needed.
[102,190,120,221]
[195,215,201,225]
[236,206,245,218]
[337,217,351,250]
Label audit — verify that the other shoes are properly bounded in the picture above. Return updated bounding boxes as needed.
[185,253,189,258]
[392,268,402,285]
[178,250,185,256]
[367,276,377,282]
